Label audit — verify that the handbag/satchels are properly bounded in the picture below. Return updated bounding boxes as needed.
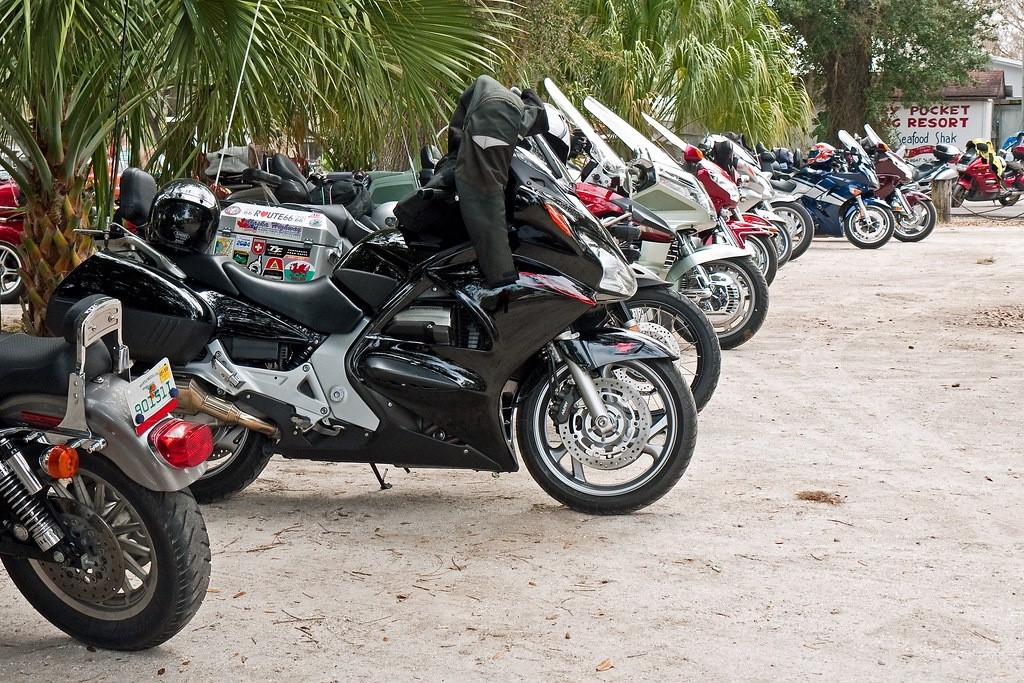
[308,180,356,209]
[345,182,371,220]
[205,145,265,176]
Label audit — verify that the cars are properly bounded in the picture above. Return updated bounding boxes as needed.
[0,169,37,303]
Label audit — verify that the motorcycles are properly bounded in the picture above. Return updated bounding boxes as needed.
[0,294,209,652]
[45,130,700,518]
[216,77,1023,433]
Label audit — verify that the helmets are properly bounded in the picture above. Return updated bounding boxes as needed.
[145,177,221,256]
[806,142,836,165]
[370,201,399,230]
[350,170,365,181]
[861,136,876,156]
